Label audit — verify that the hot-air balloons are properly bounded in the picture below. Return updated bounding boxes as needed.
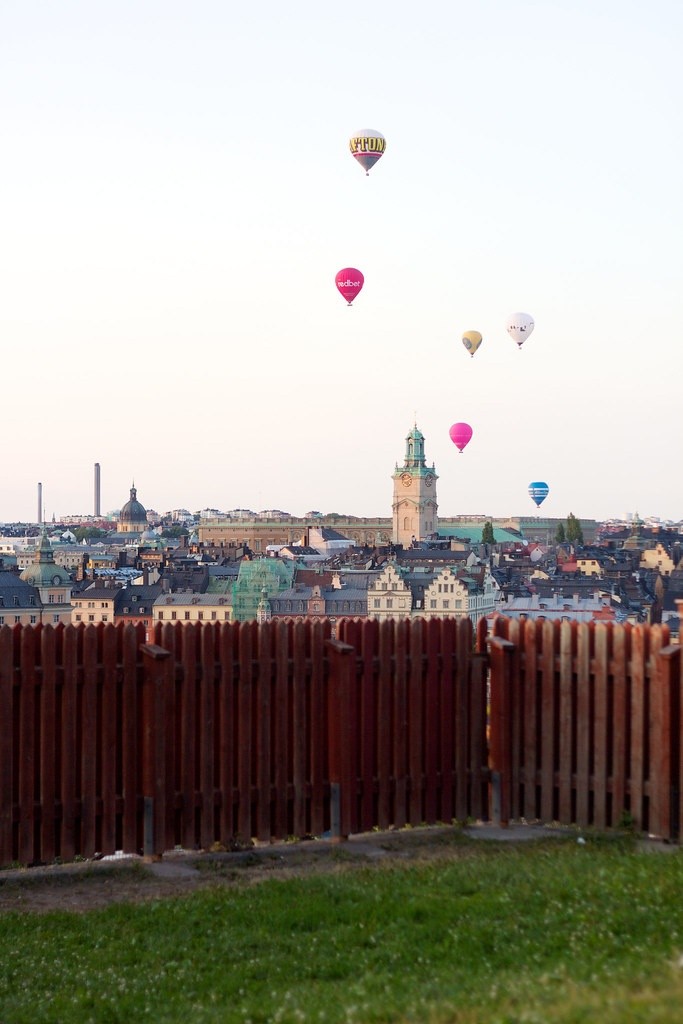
[350,130,387,177]
[505,312,535,350]
[528,481,549,509]
[449,422,473,454]
[462,329,483,358]
[335,266,365,307]
